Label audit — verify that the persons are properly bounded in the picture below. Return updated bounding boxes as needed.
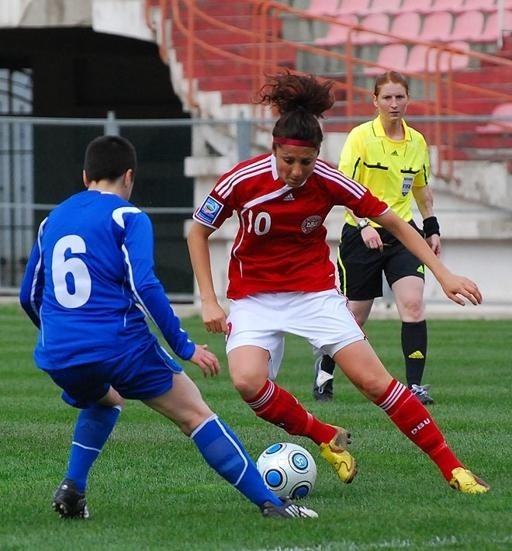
[17,131,323,521]
[310,67,446,406]
[184,64,491,495]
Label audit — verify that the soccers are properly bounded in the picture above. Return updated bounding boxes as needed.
[256,442,318,503]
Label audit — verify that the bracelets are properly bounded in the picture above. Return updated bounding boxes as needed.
[422,215,441,236]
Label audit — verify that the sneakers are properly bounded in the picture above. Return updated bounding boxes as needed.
[318,423,360,484]
[312,356,334,401]
[52,478,89,519]
[449,467,491,494]
[408,386,436,405]
[260,495,319,521]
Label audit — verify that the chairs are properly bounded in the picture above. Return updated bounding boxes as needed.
[302,0,512,138]
